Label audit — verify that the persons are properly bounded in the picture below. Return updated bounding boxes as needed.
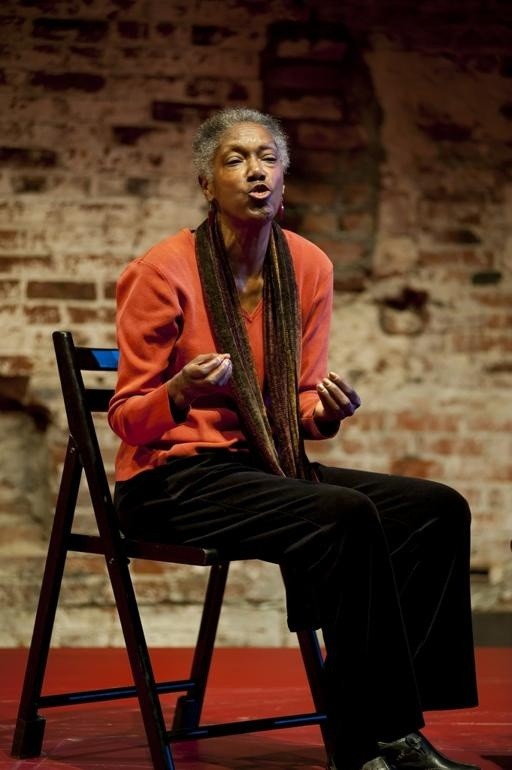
[107,107,479,770]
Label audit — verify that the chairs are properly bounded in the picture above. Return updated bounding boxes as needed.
[10,331,345,770]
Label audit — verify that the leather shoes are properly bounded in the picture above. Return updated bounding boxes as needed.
[325,731,481,770]
